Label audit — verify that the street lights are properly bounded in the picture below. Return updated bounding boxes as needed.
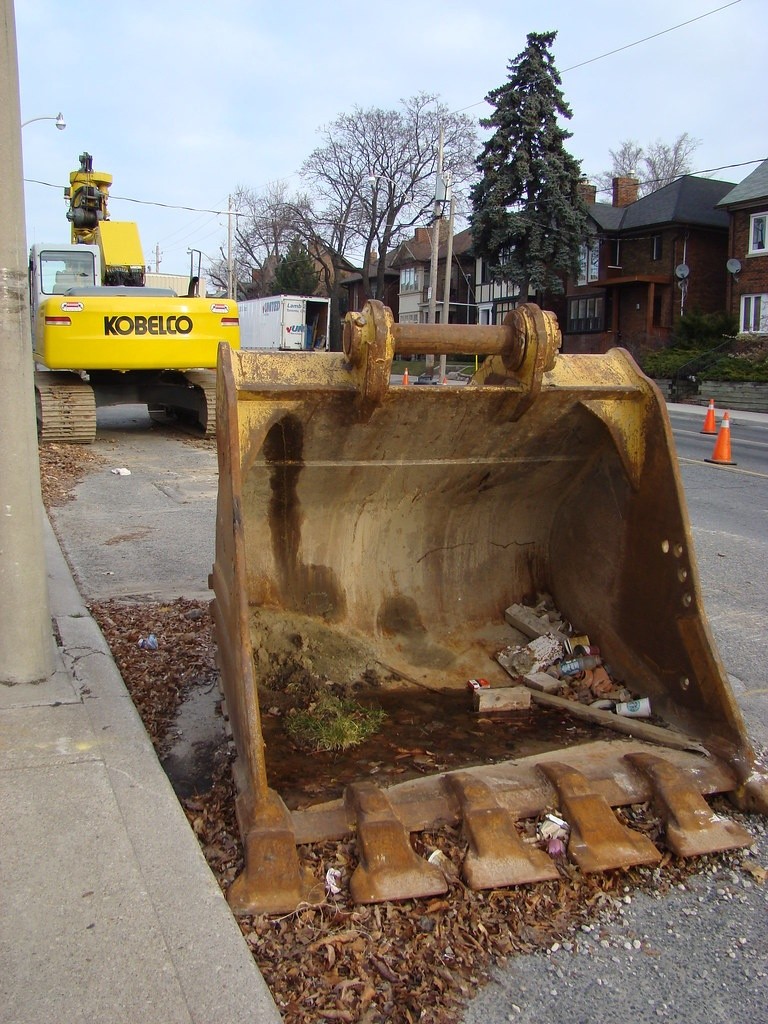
[368,175,438,378]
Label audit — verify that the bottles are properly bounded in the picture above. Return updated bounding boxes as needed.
[558,657,601,675]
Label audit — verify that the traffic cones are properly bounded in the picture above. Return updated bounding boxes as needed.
[401,368,409,385]
[703,411,738,465]
[699,398,719,435]
[441,377,447,385]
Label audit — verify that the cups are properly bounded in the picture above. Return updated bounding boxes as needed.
[574,643,600,657]
[564,635,590,655]
[616,697,652,718]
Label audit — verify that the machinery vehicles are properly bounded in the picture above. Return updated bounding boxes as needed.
[26,151,243,446]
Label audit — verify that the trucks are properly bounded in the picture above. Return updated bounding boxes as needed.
[235,294,332,354]
